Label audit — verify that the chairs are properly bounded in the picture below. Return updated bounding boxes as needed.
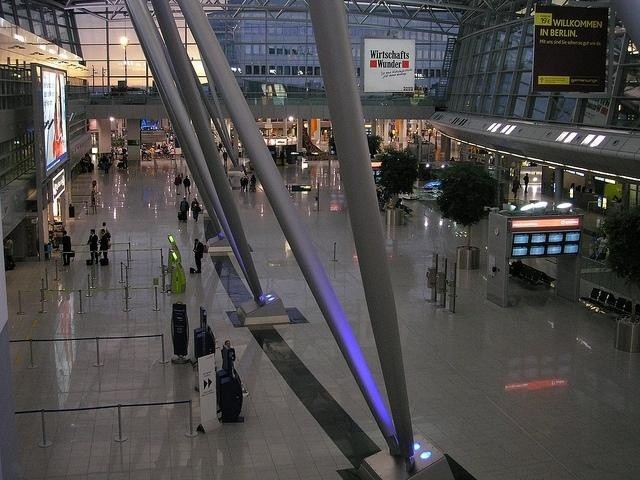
[510,262,552,290]
[579,286,639,321]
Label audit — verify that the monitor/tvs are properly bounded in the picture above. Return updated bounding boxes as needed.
[511,231,581,256]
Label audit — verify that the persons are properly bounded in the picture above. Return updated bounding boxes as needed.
[190,197,200,223]
[450,156,455,162]
[4,236,16,271]
[98,228,108,259]
[428,126,434,142]
[218,141,224,153]
[243,173,250,192]
[396,198,413,215]
[175,174,183,195]
[184,176,192,194]
[389,131,393,142]
[595,233,608,260]
[99,221,112,257]
[179,197,189,224]
[251,174,257,192]
[512,176,522,198]
[193,238,204,273]
[62,230,72,266]
[86,228,98,264]
[220,339,236,370]
[523,172,530,193]
[240,177,244,192]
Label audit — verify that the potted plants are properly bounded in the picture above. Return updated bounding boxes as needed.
[374,144,426,227]
[437,159,501,269]
[593,196,639,355]
